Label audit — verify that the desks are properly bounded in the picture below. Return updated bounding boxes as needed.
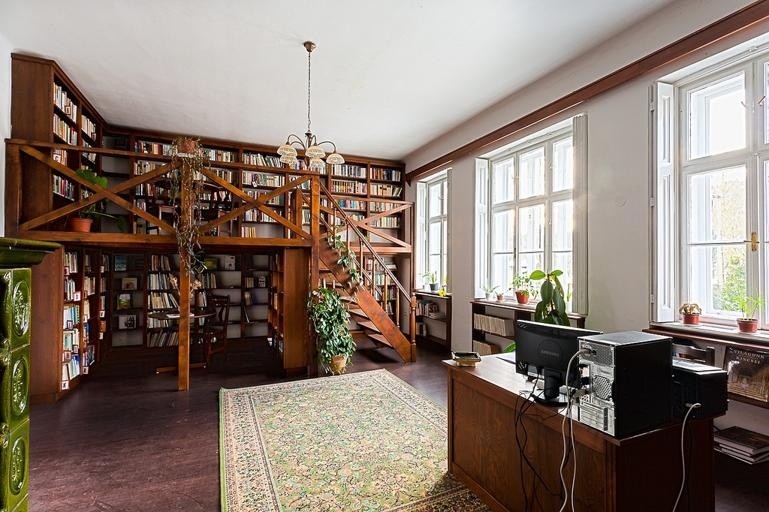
[440,352,726,512]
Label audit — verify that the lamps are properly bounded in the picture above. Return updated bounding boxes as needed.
[277,43,345,169]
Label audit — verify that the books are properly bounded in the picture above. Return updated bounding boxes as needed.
[471,310,515,356]
[133,137,237,235]
[52,85,113,390]
[241,154,402,240]
[353,257,398,313]
[115,254,236,348]
[496,354,516,364]
[245,276,283,351]
[415,298,445,336]
[712,425,769,466]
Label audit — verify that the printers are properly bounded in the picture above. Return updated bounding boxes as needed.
[672,358,728,419]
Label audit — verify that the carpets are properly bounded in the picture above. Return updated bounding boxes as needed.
[218,368,491,512]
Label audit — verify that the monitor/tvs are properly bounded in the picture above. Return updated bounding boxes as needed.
[515,320,602,407]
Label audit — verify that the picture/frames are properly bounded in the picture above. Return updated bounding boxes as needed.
[724,345,769,410]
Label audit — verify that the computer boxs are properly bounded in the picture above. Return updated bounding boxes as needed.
[575,330,673,439]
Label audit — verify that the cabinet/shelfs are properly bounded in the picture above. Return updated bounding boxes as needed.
[470,302,585,352]
[26,239,406,400]
[13,54,407,249]
[416,292,452,351]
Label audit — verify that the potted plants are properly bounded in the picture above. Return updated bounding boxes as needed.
[422,270,438,291]
[508,266,539,304]
[482,285,500,299]
[679,302,703,325]
[733,289,766,332]
[495,288,506,301]
